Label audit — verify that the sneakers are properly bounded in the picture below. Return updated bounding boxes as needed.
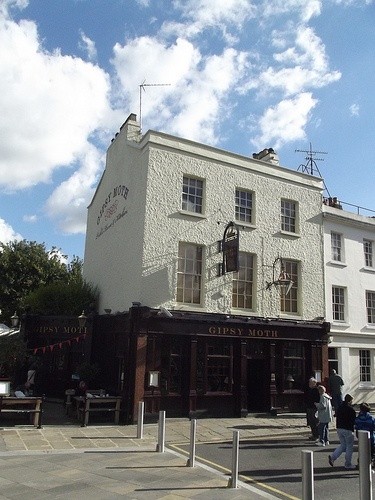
[315,442,325,447]
[325,441,329,446]
[328,455,334,467]
[345,464,356,469]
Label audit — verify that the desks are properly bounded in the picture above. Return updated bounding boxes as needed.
[73,396,125,425]
[0,394,46,427]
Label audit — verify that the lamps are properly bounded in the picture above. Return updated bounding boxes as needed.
[266,252,294,296]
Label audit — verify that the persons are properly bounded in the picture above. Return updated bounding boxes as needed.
[352,403,375,469]
[303,377,320,441]
[313,385,333,447]
[75,380,86,398]
[316,369,344,409]
[16,381,30,397]
[328,394,356,470]
